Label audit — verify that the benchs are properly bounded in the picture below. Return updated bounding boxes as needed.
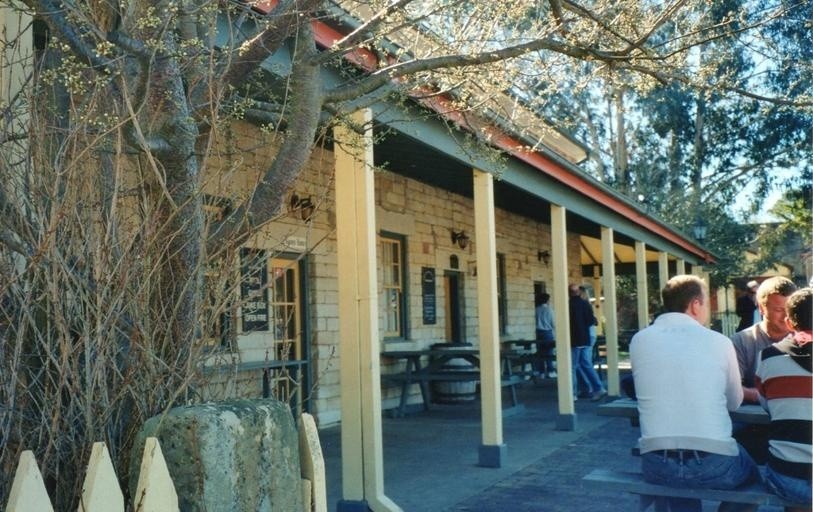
[382,356,608,419]
[582,469,812,512]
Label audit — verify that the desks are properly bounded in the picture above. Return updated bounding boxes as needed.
[500,341,557,386]
[598,397,771,428]
[382,351,518,418]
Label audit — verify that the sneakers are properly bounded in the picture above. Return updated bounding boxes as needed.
[573,388,608,401]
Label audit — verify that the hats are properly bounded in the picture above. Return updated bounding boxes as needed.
[746,280,760,294]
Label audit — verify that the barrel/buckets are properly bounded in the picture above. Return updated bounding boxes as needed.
[431,343,476,405]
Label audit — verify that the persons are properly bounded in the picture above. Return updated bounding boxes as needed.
[536,283,608,402]
[729,276,812,507]
[629,274,761,491]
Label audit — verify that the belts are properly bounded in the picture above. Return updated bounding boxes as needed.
[632,448,710,459]
